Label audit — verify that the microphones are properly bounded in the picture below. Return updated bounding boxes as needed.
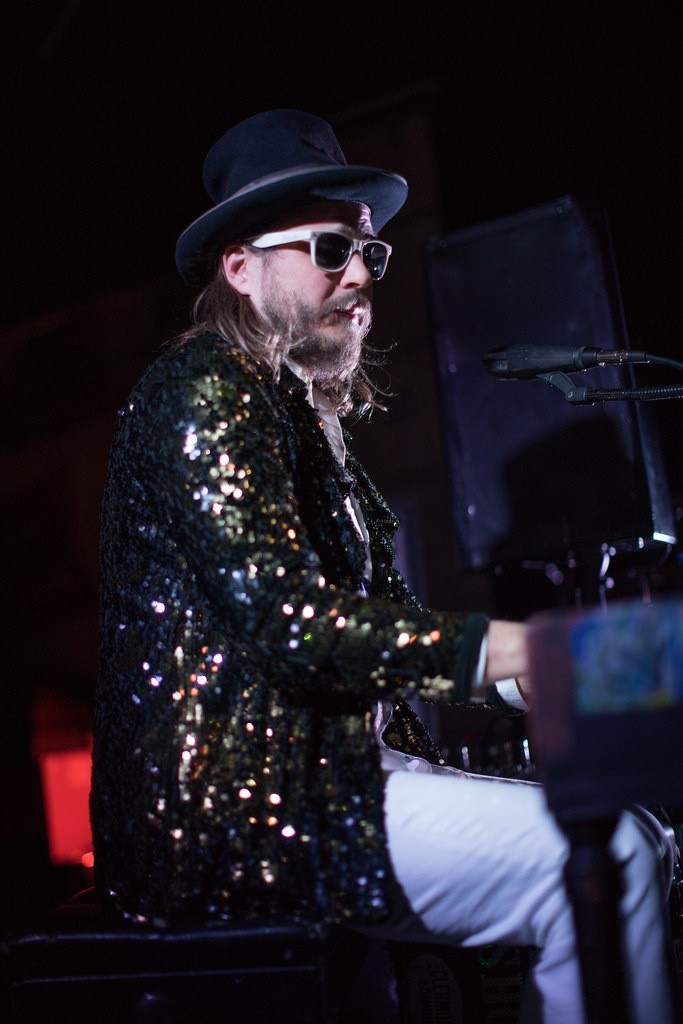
[480,343,649,383]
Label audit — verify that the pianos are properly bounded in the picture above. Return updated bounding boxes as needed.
[519,525,683,1024]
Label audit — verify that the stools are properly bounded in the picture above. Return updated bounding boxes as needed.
[0,905,333,1024]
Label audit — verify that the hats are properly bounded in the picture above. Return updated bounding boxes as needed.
[176,108,410,288]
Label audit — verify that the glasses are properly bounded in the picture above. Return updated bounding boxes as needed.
[243,229,391,281]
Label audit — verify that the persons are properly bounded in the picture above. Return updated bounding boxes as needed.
[91,109,683,1024]
